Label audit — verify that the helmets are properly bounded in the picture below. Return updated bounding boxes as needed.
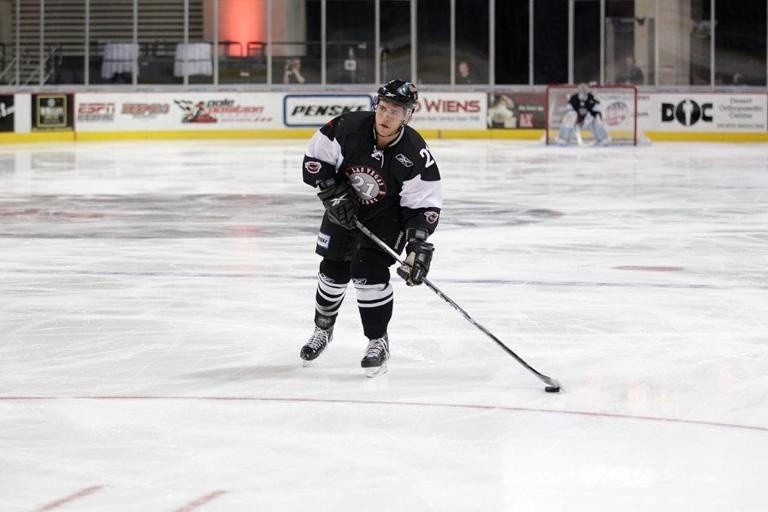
[377,79,418,120]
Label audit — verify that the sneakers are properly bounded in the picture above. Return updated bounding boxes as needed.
[301,325,333,360]
[361,334,389,367]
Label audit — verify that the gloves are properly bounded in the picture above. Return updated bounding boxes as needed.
[318,181,358,230]
[397,242,434,286]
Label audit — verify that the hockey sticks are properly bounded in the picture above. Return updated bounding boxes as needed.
[566,93,598,147]
[354,220,560,392]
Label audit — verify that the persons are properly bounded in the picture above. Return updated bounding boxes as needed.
[456,61,479,84]
[615,57,643,84]
[298,79,443,368]
[556,83,611,147]
[282,56,310,84]
